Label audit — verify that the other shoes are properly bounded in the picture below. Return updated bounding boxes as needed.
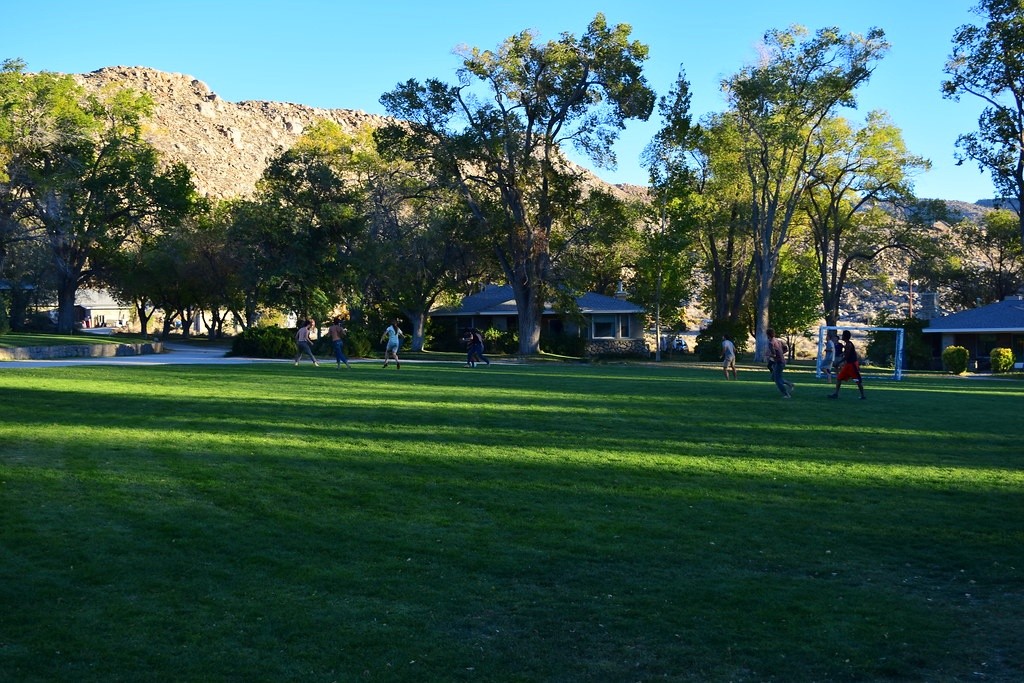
[396,362,401,370]
[782,394,791,399]
[827,392,839,398]
[382,363,388,368]
[789,383,794,392]
[315,362,319,366]
[294,362,298,366]
[855,395,867,400]
[463,364,472,367]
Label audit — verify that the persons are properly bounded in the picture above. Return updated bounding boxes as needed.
[294,320,320,367]
[326,317,351,370]
[462,328,490,368]
[819,330,866,400]
[379,319,408,370]
[766,328,795,398]
[719,334,737,381]
